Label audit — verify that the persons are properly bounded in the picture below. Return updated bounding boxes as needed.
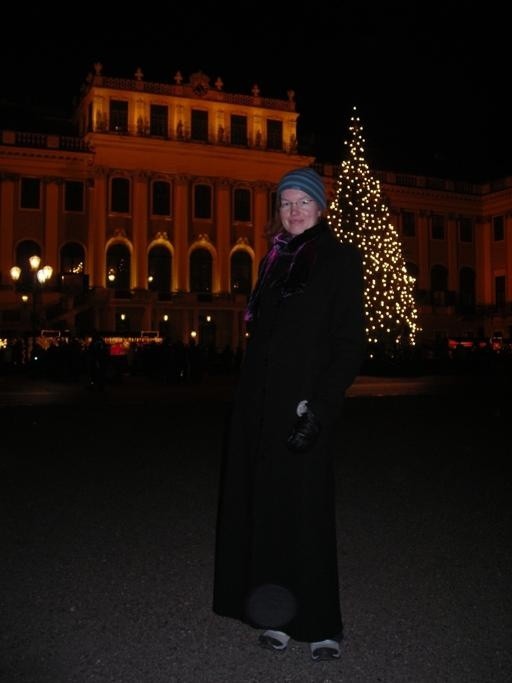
[363,335,510,376]
[413,286,457,307]
[2,333,243,377]
[209,163,358,659]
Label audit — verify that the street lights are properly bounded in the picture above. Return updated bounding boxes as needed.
[6,251,57,370]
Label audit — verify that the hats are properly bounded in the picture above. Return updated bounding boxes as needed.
[276,168,328,210]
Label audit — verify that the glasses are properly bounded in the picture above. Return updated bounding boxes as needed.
[279,198,314,211]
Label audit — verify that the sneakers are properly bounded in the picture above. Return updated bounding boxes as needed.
[259,629,292,650]
[309,639,342,661]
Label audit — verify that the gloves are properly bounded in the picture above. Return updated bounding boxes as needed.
[286,410,322,455]
